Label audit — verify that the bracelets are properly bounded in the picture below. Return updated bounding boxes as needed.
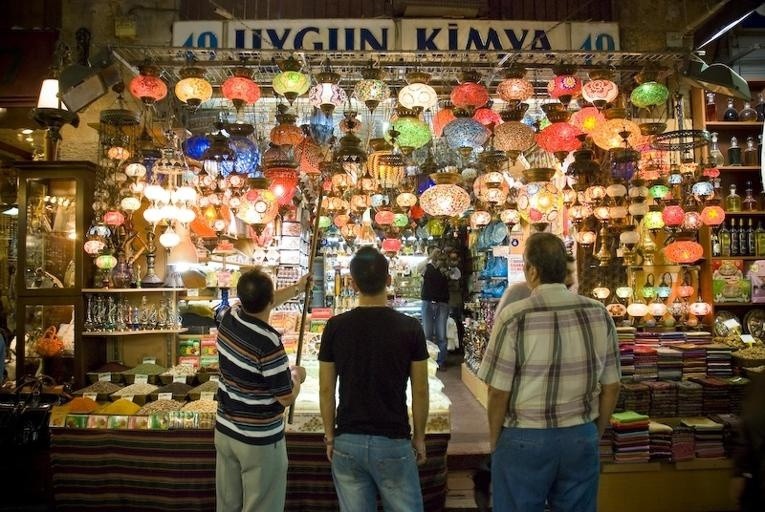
[323,435,335,446]
[292,284,300,298]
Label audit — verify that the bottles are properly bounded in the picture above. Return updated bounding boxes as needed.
[83,296,184,332]
[707,91,764,257]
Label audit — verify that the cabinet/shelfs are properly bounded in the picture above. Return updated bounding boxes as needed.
[690,83,765,335]
[79,286,188,336]
[0,402,56,512]
[0,159,107,399]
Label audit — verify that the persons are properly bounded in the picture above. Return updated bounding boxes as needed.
[212,270,315,511]
[471,228,623,512]
[488,250,577,324]
[315,244,433,512]
[416,245,462,372]
[726,360,765,512]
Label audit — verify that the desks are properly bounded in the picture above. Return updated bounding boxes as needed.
[46,384,452,511]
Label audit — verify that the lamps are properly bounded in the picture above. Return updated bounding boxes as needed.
[83,51,727,326]
[27,39,79,161]
